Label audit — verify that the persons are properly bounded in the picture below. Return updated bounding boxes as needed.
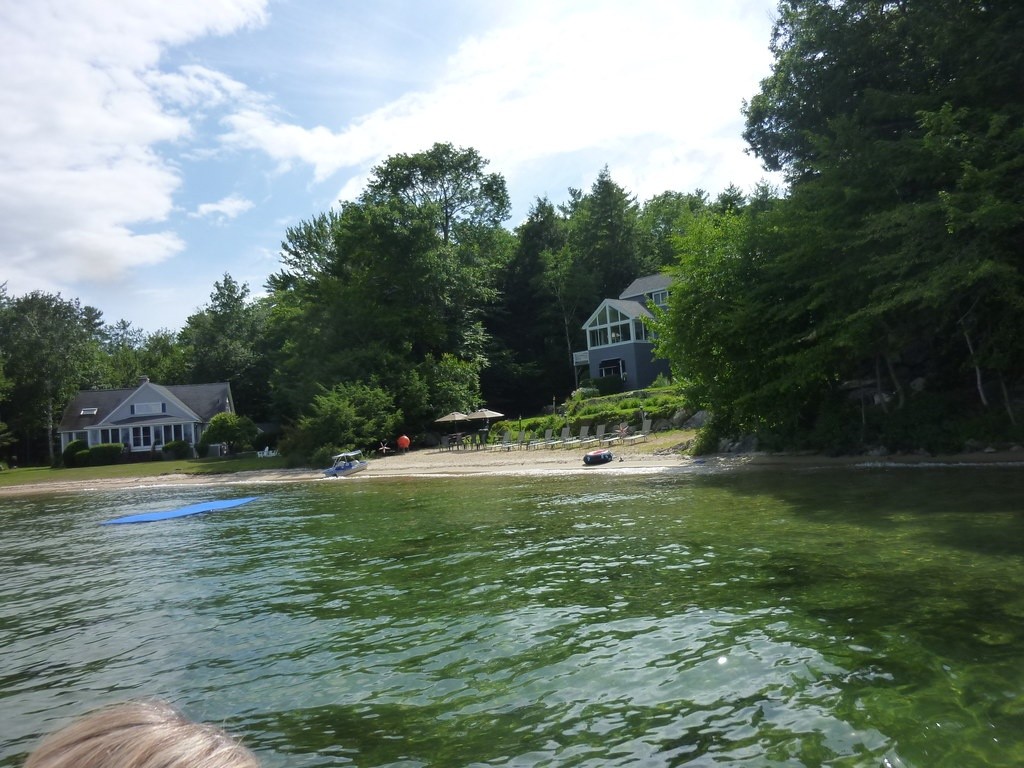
[447,432,481,451]
[23,692,263,768]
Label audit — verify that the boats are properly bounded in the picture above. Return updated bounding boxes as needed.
[323,448,369,479]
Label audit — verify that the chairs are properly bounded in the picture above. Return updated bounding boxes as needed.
[439,419,657,451]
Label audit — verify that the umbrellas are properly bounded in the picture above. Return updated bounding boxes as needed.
[465,408,504,420]
[434,411,470,422]
